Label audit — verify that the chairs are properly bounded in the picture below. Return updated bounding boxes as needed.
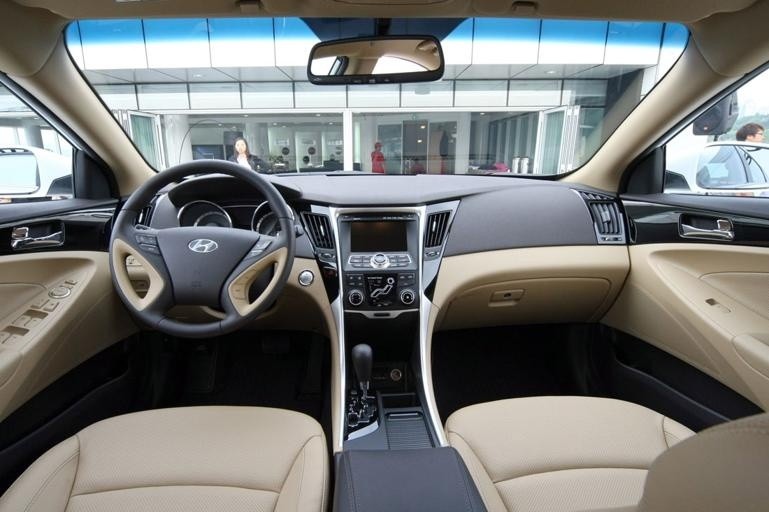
[446,394,768,510]
[2,405,328,509]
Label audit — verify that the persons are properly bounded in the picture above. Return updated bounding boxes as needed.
[736,122,765,143]
[411,158,426,175]
[228,136,267,173]
[371,142,385,174]
